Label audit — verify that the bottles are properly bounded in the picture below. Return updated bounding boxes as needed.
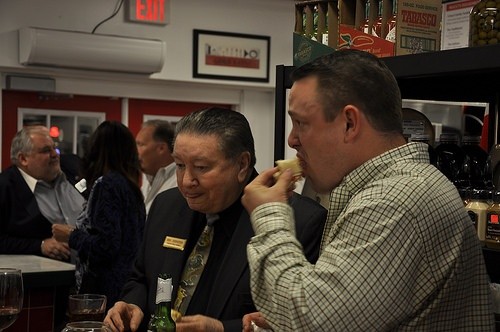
[371,0,382,38]
[385,0,397,44]
[468,0,500,47]
[358,0,370,34]
[409,132,500,250]
[146,271,177,332]
[309,5,319,41]
[321,10,328,46]
[300,7,307,37]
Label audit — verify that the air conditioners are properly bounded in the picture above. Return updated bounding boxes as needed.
[19,26,167,76]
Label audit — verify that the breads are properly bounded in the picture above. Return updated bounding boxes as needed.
[273,158,303,187]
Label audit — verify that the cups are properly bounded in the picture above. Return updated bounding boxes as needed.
[62,321,114,332]
[0,267,23,332]
[69,294,107,322]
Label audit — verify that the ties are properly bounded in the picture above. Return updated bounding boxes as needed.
[174,214,219,316]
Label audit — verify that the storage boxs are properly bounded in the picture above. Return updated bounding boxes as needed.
[292,0,441,66]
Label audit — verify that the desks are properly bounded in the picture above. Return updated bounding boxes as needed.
[0,254,76,332]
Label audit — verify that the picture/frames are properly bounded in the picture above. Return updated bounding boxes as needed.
[192,27,271,82]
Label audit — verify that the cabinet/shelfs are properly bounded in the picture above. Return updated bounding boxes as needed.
[273,45,499,285]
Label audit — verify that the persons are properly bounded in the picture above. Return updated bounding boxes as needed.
[51,119,147,323]
[100,107,328,332]
[0,125,90,332]
[236,49,495,332]
[135,121,180,218]
